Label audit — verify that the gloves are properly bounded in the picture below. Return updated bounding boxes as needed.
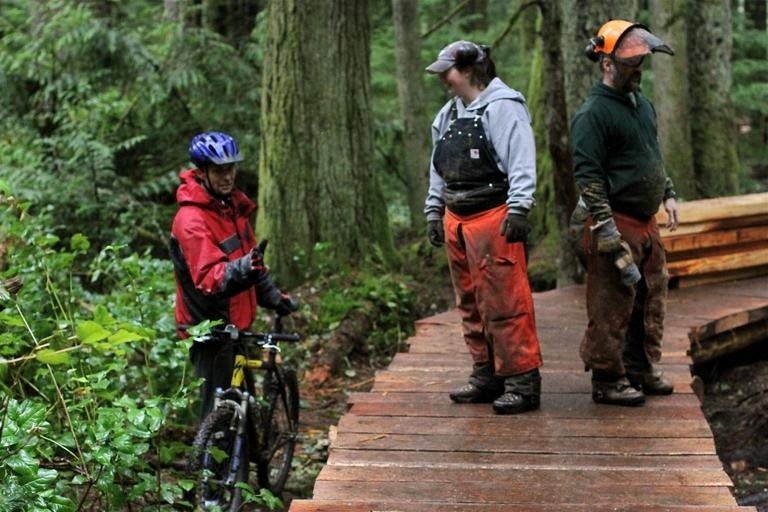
[426,219,445,247]
[613,243,641,286]
[589,216,621,256]
[498,211,531,242]
[240,237,269,277]
[275,292,300,317]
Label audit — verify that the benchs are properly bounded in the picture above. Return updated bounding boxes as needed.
[655,192,768,288]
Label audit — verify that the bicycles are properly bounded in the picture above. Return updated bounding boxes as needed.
[176,292,300,512]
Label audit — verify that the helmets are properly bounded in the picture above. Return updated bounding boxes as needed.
[585,19,675,63]
[188,130,244,170]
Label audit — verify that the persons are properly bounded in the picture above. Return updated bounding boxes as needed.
[569,19,681,405]
[423,40,543,413]
[170,132,298,512]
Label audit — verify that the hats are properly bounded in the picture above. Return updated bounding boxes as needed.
[425,41,485,73]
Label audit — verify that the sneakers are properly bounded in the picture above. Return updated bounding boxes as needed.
[627,369,673,394]
[592,376,646,405]
[494,388,541,414]
[449,381,505,403]
